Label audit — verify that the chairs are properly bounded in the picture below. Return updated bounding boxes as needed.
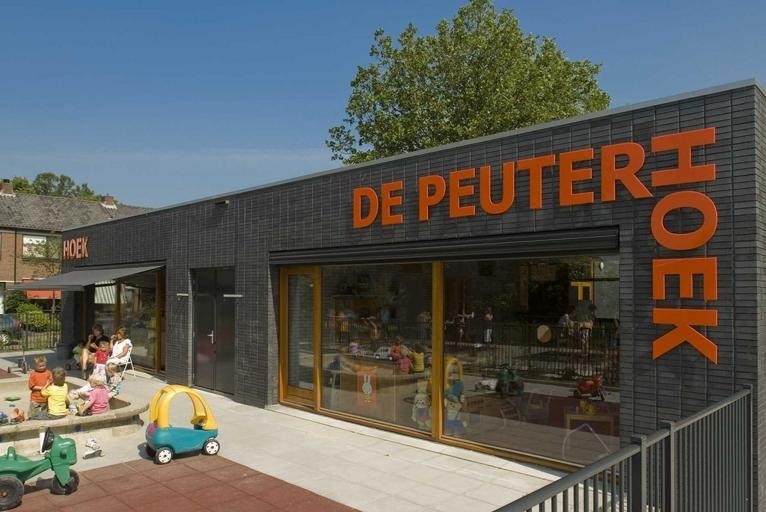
[113,344,136,380]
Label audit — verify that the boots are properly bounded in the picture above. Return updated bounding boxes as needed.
[80,368,88,380]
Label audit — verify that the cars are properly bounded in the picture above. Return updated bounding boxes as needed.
[0,314,22,345]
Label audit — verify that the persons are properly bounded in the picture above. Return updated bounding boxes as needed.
[28,324,133,419]
[320,301,620,385]
[142,333,156,367]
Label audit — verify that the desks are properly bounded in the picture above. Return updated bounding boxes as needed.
[564,405,621,473]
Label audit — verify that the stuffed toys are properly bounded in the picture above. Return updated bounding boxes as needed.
[411,390,469,438]
